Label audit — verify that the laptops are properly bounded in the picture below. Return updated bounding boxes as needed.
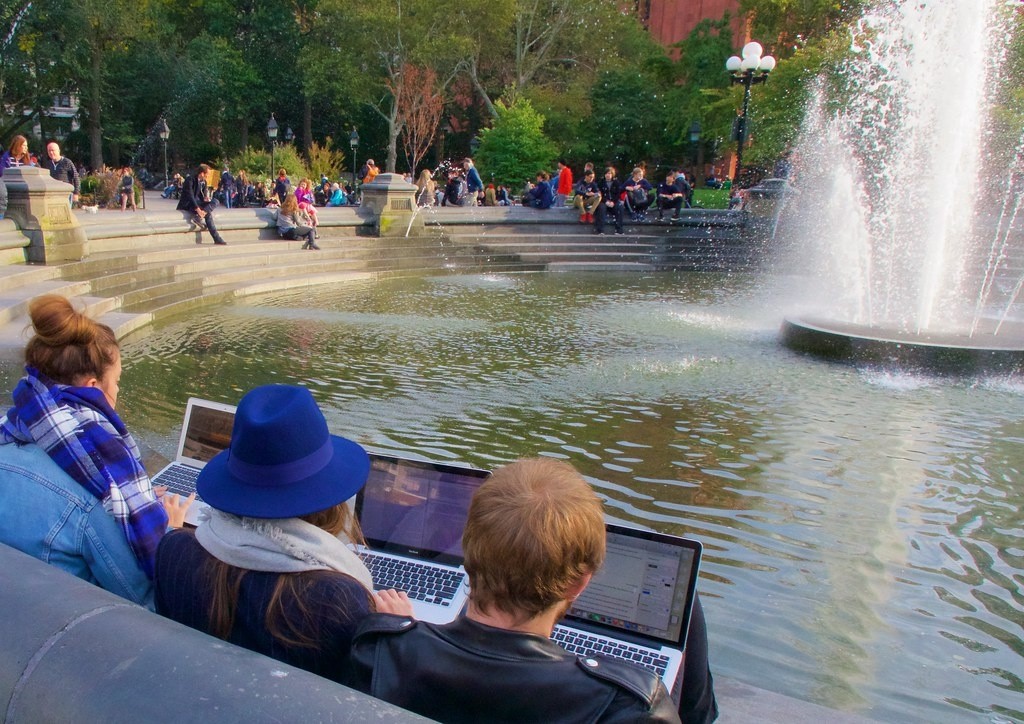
[547,522,704,696]
[344,452,494,625]
[149,397,238,527]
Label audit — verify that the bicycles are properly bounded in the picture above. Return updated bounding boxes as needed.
[684,198,703,209]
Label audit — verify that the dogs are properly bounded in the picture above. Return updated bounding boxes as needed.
[81,204,100,214]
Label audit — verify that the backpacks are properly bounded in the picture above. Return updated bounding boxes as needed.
[280,181,291,192]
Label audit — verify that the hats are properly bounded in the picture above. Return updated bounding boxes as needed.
[279,169,286,175]
[367,159,374,164]
[196,384,371,518]
[333,183,339,188]
[315,186,321,190]
[460,157,473,163]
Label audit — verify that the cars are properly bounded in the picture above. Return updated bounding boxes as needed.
[748,180,803,199]
[705,176,722,188]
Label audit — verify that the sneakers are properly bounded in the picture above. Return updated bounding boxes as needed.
[580,212,593,223]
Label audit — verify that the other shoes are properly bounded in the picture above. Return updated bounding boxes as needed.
[215,238,226,245]
[658,216,664,222]
[598,231,605,236]
[190,217,205,229]
[670,215,680,221]
[133,206,136,212]
[614,230,625,236]
[631,214,644,223]
[161,194,167,198]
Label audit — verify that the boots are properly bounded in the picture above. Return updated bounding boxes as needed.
[308,231,320,250]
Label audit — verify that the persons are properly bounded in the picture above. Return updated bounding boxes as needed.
[157,384,415,683]
[0,134,793,251]
[1,293,196,613]
[350,458,718,724]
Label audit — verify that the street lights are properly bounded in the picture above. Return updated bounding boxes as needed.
[350,126,360,204]
[159,120,172,188]
[268,113,279,182]
[724,40,775,210]
